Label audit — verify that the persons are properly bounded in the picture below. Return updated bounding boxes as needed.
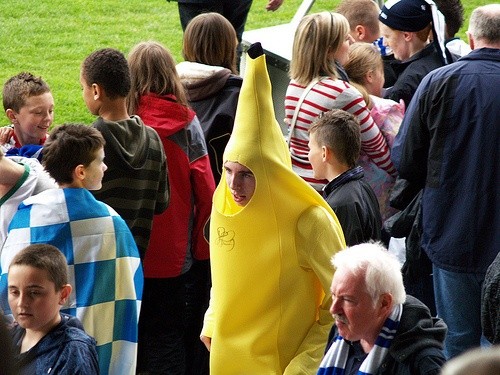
[431,0,473,62]
[305,110,385,247]
[0,71,56,156]
[318,240,449,375]
[333,0,397,56]
[0,144,61,252]
[165,0,283,74]
[282,11,406,193]
[0,243,99,375]
[1,122,146,374]
[480,252,500,351]
[172,11,245,375]
[383,0,464,112]
[341,40,406,230]
[389,2,500,365]
[1,49,171,261]
[124,40,217,375]
[200,41,348,375]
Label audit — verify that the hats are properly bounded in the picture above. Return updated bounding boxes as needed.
[378,0,432,32]
